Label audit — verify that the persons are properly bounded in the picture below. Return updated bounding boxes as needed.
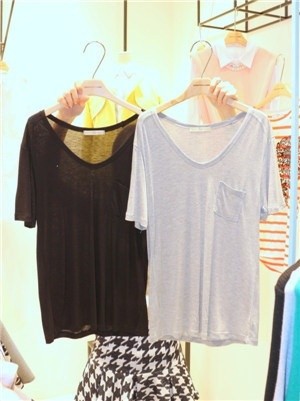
[49,77,240,401]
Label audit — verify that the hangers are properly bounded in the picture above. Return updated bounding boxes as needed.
[250,54,298,119]
[141,39,265,124]
[27,42,144,121]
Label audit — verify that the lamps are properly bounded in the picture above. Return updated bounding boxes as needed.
[0,0,14,72]
[226,0,246,48]
